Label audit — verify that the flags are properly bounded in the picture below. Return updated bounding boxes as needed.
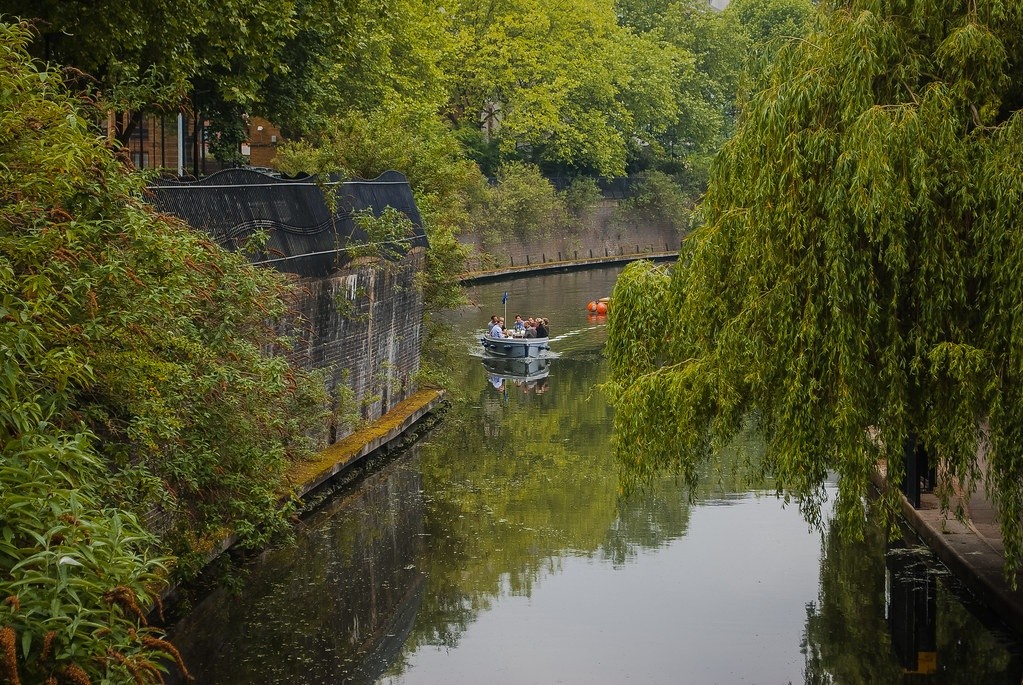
[502,291,508,304]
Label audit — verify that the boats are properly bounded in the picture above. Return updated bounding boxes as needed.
[481,331,551,358]
[588,296,615,313]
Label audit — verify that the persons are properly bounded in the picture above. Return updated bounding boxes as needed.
[513,314,549,339]
[488,316,509,339]
